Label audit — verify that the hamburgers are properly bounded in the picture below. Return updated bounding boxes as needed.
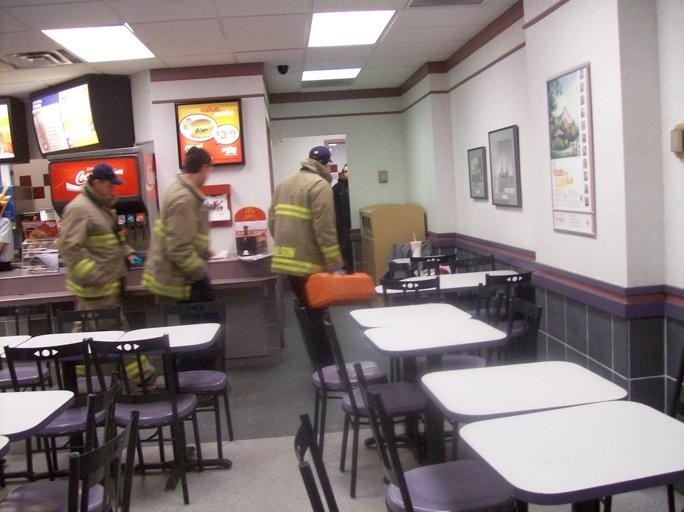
[191,118,212,138]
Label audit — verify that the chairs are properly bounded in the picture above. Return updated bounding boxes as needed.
[455,255,495,273]
[0,302,59,451]
[468,282,509,319]
[295,413,339,512]
[67,410,140,512]
[57,305,121,393]
[291,298,387,460]
[381,278,442,306]
[3,338,113,481]
[486,271,534,315]
[412,298,544,376]
[355,362,519,511]
[486,297,543,367]
[410,255,450,276]
[89,333,189,505]
[322,309,428,498]
[596,347,684,512]
[155,298,232,460]
[0,371,119,512]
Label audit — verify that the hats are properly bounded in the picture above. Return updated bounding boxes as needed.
[310,145,333,164]
[93,163,122,186]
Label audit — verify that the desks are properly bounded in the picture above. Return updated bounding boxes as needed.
[420,360,628,462]
[364,318,507,465]
[458,400,684,512]
[117,321,232,474]
[374,269,523,306]
[350,302,472,464]
[0,335,32,489]
[392,254,447,275]
[2,330,126,480]
[0,390,74,441]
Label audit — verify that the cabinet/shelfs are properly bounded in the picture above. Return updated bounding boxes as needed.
[18,208,61,266]
[359,203,427,285]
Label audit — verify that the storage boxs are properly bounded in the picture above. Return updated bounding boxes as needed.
[307,271,375,309]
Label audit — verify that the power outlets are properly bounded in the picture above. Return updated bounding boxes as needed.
[671,123,684,161]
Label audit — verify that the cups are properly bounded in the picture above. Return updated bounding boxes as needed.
[410,241,421,260]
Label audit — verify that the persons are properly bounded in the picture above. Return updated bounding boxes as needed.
[332,163,354,272]
[0,204,15,274]
[141,147,220,406]
[56,164,165,390]
[268,146,346,373]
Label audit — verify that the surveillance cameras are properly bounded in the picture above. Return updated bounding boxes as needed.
[278,64,289,76]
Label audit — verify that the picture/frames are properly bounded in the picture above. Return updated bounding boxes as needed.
[488,125,523,209]
[545,61,597,239]
[467,146,488,199]
